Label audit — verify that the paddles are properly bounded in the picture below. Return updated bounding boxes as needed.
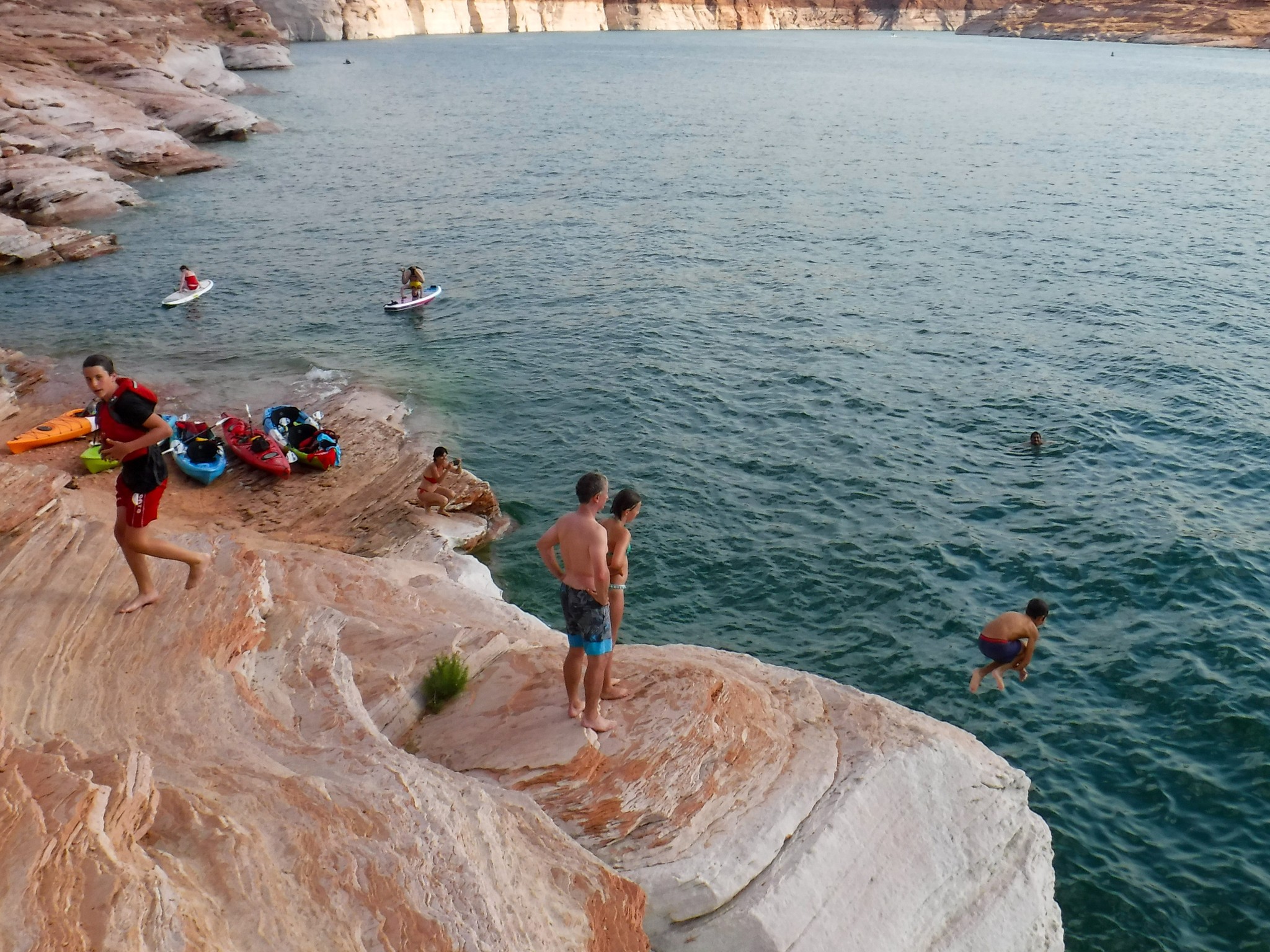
[244,403,255,439]
[278,417,298,463]
[161,416,230,456]
[312,410,325,453]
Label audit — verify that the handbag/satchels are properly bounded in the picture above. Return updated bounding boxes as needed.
[250,437,269,453]
[187,437,224,464]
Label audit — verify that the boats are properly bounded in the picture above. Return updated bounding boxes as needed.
[383,284,442,312]
[262,405,341,470]
[80,414,180,475]
[221,412,291,479]
[162,278,214,307]
[168,419,227,486]
[6,407,98,455]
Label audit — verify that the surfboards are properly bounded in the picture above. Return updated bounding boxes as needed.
[162,279,214,306]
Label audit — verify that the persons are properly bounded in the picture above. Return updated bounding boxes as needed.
[82,354,210,613]
[536,473,618,731]
[398,266,425,299]
[179,265,204,293]
[597,489,641,699]
[1021,432,1052,445]
[969,598,1049,694]
[417,446,462,517]
